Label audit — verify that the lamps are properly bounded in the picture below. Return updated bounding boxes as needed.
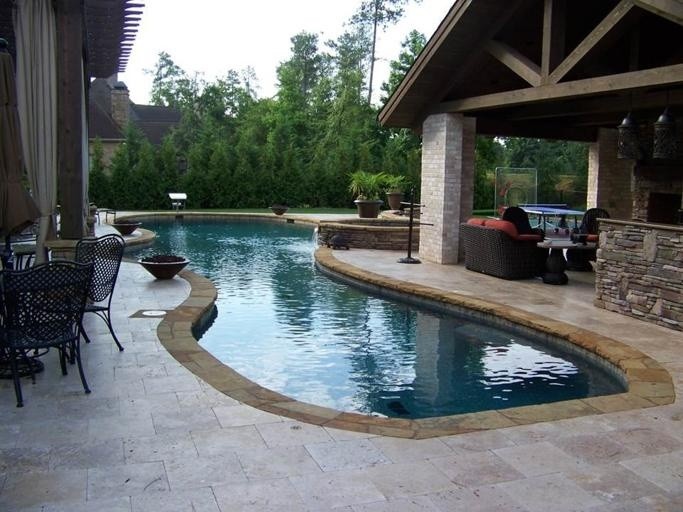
[615,109,671,162]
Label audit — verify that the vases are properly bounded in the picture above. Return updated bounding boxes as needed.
[141,252,192,281]
[115,217,143,235]
[270,201,289,217]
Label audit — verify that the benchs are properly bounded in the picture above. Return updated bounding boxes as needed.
[94,207,117,224]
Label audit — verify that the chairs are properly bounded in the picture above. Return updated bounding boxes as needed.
[460,206,613,286]
[1,231,128,411]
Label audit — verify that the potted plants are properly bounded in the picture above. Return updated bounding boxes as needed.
[348,171,409,219]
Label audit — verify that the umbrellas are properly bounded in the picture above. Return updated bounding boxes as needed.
[0,37,44,269]
